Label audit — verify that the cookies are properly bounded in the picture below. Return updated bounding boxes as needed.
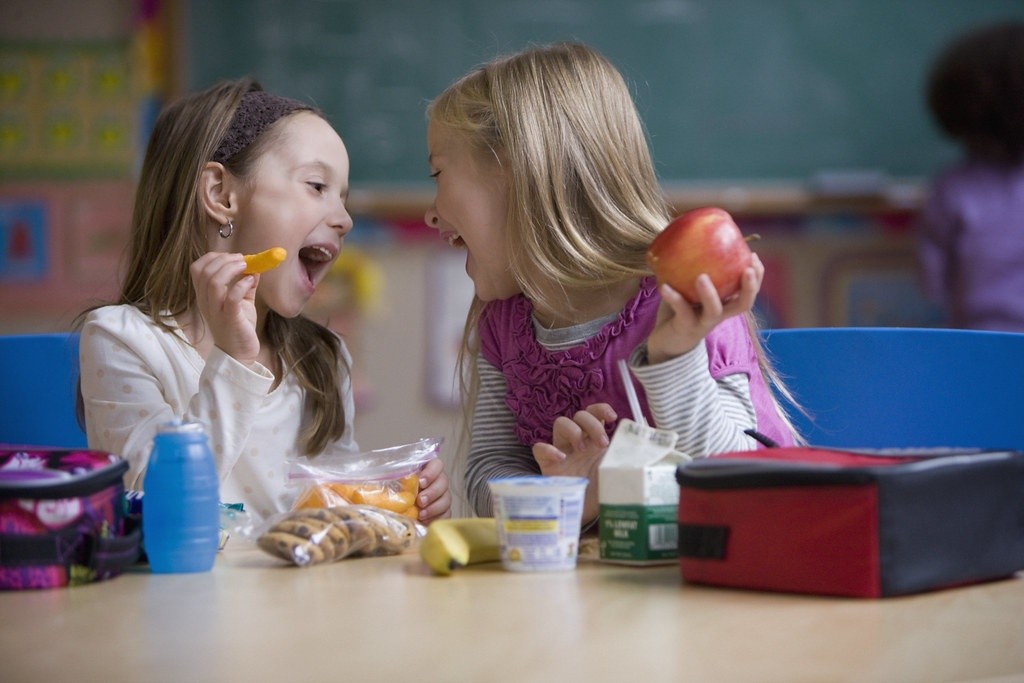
[257,506,411,561]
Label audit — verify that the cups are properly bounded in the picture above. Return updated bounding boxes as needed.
[488,476,590,572]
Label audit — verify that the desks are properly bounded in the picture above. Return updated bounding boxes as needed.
[0,538,1024,683]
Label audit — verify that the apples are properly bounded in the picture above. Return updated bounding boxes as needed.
[646,206,759,305]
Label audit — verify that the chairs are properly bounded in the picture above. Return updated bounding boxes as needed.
[758,328,1024,452]
[0,333,87,448]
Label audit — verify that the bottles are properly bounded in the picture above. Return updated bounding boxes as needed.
[143,422,218,572]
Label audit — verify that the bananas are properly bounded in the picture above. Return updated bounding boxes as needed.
[422,517,500,574]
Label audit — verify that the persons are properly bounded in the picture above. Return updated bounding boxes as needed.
[72,73,453,531]
[917,22,1024,333]
[424,43,813,535]
